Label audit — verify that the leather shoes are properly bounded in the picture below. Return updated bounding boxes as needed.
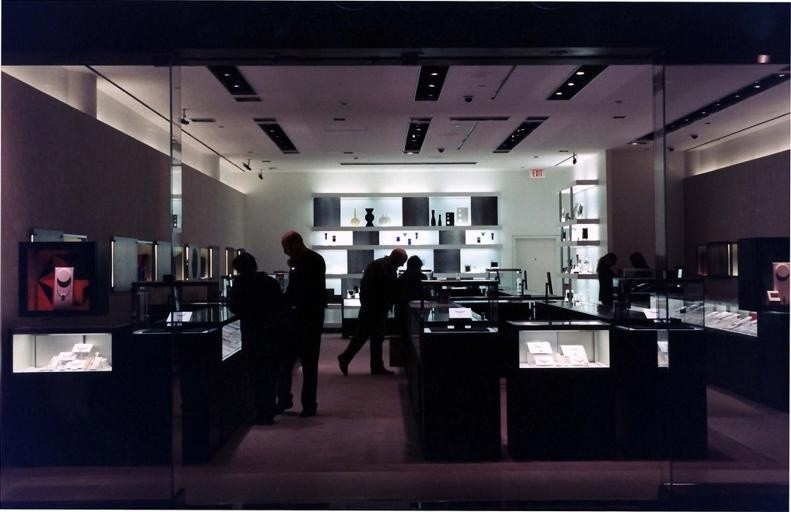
[275,403,292,409]
[370,369,395,376]
[337,354,349,376]
[298,406,317,416]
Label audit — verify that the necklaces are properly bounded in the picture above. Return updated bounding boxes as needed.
[575,358,584,363]
[569,348,583,355]
[55,283,72,300]
[778,277,789,300]
[540,357,551,362]
[58,275,71,287]
[536,346,547,352]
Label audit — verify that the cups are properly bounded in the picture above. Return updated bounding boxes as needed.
[573,294,582,307]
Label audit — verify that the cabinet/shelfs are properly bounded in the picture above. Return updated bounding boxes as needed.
[340,270,435,339]
[555,179,598,302]
[1,273,293,466]
[311,190,502,330]
[408,275,708,464]
[630,275,790,413]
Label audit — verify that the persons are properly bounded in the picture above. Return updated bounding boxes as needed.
[597,252,618,305]
[337,249,407,376]
[393,255,431,318]
[630,252,652,277]
[276,230,326,418]
[223,251,293,423]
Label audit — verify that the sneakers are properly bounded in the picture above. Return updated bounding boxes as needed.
[253,411,275,426]
